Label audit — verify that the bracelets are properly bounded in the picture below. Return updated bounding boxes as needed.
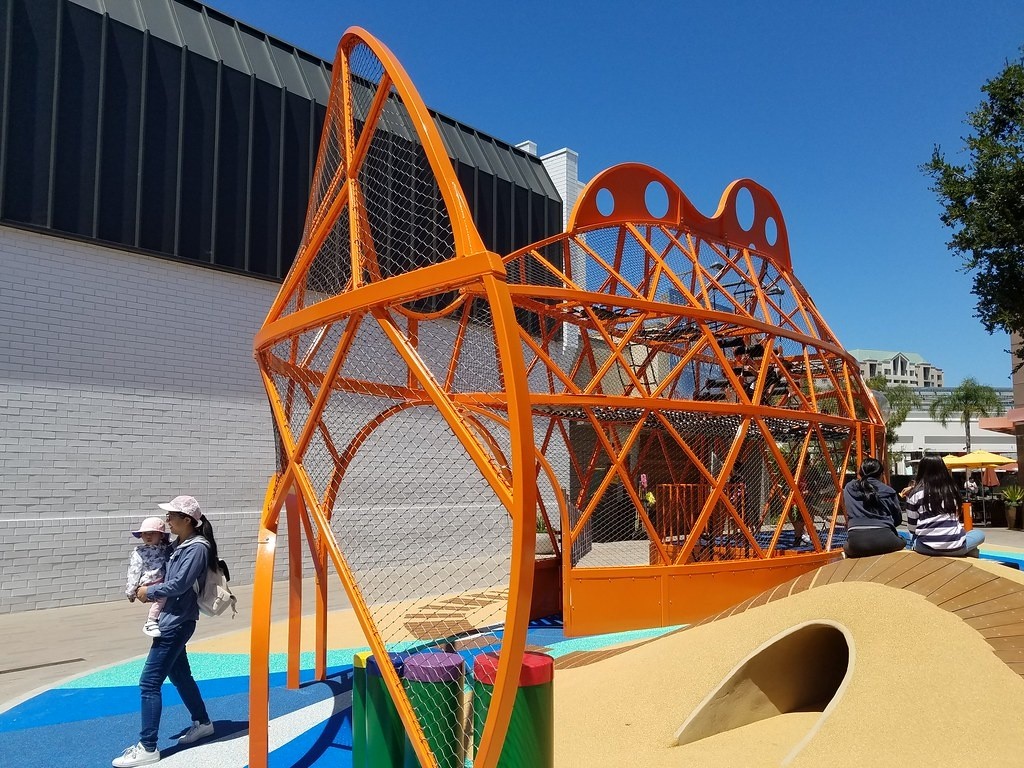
[911,486,913,489]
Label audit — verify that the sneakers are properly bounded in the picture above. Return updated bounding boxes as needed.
[142,622,161,637]
[178,720,214,744]
[112,742,160,767]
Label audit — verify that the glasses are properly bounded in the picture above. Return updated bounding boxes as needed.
[166,514,187,522]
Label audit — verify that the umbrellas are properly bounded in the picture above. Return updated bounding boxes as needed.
[946,449,1017,528]
[943,455,958,477]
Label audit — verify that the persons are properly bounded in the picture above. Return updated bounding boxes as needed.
[901,456,986,556]
[733,346,781,403]
[125,518,171,636]
[787,453,820,546]
[844,458,907,559]
[909,480,915,486]
[964,477,978,493]
[111,496,217,768]
[730,462,747,534]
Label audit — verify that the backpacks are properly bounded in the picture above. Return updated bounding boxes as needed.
[185,538,238,620]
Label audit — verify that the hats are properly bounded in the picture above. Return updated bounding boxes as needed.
[157,495,202,526]
[132,518,171,542]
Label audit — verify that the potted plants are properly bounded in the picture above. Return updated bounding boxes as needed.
[535,511,558,554]
[1000,483,1024,530]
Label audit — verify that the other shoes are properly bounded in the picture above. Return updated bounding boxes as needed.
[801,535,811,546]
[794,536,800,546]
